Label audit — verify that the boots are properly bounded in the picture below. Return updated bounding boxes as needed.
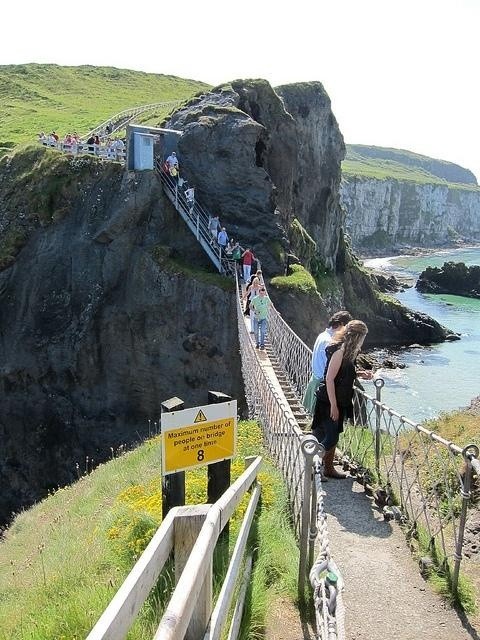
[311,467,327,482]
[324,444,346,479]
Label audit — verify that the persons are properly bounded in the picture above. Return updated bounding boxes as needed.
[206,213,280,351]
[301,311,354,442]
[36,121,124,161]
[163,160,170,178]
[172,175,188,191]
[166,151,179,170]
[184,186,195,215]
[311,317,375,482]
[169,163,179,187]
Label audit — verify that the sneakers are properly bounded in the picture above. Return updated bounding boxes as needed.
[256,344,259,349]
[250,332,254,334]
[260,346,264,350]
